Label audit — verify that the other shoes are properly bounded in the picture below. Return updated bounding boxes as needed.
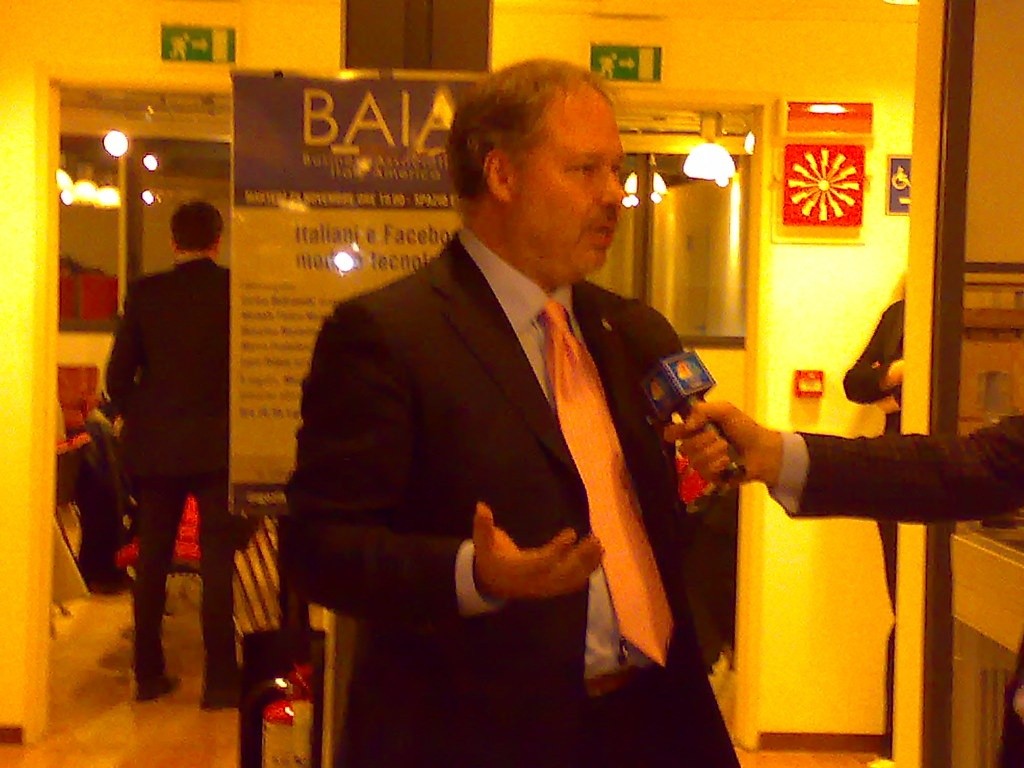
[199,668,240,710]
[134,666,179,701]
[79,564,130,593]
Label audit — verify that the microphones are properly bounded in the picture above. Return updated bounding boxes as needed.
[617,303,745,482]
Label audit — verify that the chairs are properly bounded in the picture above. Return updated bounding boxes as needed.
[55,254,324,647]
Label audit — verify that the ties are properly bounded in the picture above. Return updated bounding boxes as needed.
[533,298,677,669]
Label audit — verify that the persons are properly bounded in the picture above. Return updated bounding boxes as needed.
[640,343,1024,768]
[107,203,244,711]
[283,59,743,768]
[843,299,905,759]
[56,366,133,593]
